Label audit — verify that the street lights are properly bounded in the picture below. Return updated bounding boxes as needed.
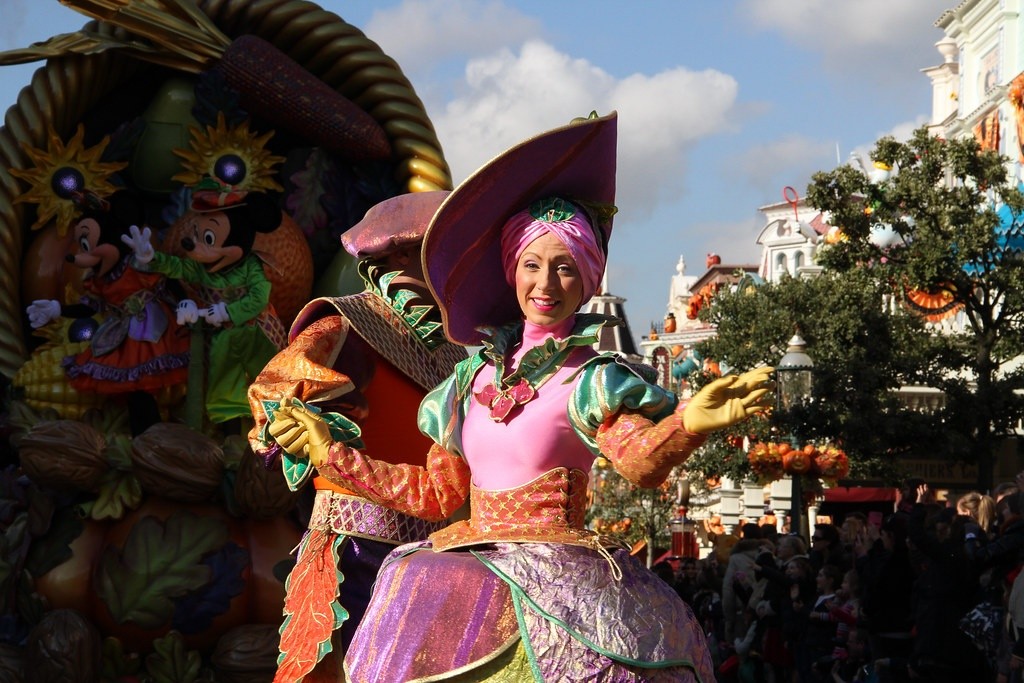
[775,330,814,530]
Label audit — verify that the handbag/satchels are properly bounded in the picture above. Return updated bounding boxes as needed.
[958,602,1005,656]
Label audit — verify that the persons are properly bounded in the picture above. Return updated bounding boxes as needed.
[278,185,774,683]
[247,192,473,683]
[651,465,1024,683]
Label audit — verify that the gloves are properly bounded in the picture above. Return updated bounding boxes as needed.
[273,405,335,469]
[683,367,776,434]
[268,396,309,458]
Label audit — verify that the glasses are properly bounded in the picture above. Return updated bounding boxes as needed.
[812,535,824,542]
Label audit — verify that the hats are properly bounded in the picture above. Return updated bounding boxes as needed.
[421,110,619,348]
[501,197,604,313]
[341,191,450,261]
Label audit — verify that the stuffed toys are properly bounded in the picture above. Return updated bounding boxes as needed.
[26,201,193,431]
[121,190,290,468]
